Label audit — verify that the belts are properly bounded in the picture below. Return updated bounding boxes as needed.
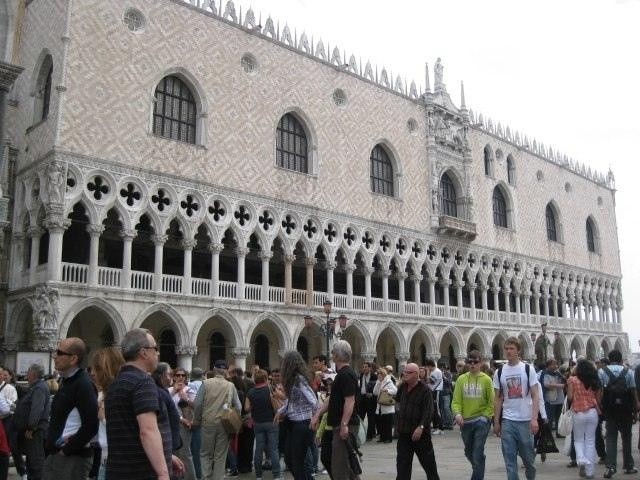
[46,448,93,455]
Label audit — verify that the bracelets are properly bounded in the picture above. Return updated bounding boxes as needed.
[187,397,190,402]
[180,416,183,423]
[341,422,348,425]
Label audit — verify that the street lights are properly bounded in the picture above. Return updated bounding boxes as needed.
[529,322,563,368]
[304,299,350,368]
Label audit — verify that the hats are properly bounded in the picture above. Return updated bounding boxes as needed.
[213,360,228,369]
[384,365,393,371]
[190,368,203,381]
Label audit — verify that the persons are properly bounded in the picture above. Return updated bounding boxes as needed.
[200,371,215,380]
[522,381,549,468]
[188,359,242,480]
[312,355,338,400]
[564,360,607,468]
[43,372,61,447]
[305,431,319,476]
[189,367,203,480]
[544,360,566,438]
[273,351,321,480]
[309,340,360,480]
[228,364,261,387]
[151,362,191,480]
[245,366,287,480]
[385,365,396,387]
[263,368,286,410]
[373,368,397,443]
[451,350,495,480]
[568,358,602,478]
[494,336,539,480]
[595,358,609,370]
[0,392,11,457]
[396,363,440,480]
[226,366,239,476]
[104,328,185,480]
[232,376,255,473]
[449,360,469,415]
[598,350,640,476]
[534,359,546,380]
[0,365,18,480]
[358,361,378,441]
[419,367,429,386]
[41,337,99,480]
[242,371,255,393]
[479,364,505,381]
[426,360,443,435]
[263,423,273,470]
[15,365,50,480]
[440,363,454,429]
[315,381,335,480]
[56,346,126,480]
[559,364,572,397]
[2,367,32,400]
[168,367,197,480]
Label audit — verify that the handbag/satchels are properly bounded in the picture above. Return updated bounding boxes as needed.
[178,387,201,428]
[344,431,363,474]
[537,411,559,454]
[378,382,396,405]
[432,381,442,399]
[267,383,287,422]
[558,395,573,436]
[246,416,255,428]
[221,382,243,433]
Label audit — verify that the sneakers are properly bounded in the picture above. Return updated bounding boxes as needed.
[257,476,264,480]
[275,476,284,480]
[430,428,444,435]
[604,468,617,478]
[322,469,328,474]
[580,462,586,477]
[567,458,577,467]
[521,462,526,468]
[598,455,608,464]
[624,468,638,474]
[586,461,592,479]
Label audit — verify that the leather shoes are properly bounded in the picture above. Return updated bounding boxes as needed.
[384,440,392,443]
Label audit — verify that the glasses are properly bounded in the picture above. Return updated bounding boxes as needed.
[456,365,463,368]
[56,349,76,357]
[137,346,159,352]
[402,370,414,374]
[174,374,186,377]
[469,360,480,364]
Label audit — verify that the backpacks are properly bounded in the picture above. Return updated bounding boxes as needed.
[602,365,632,419]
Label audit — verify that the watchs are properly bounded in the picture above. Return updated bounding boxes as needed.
[417,425,424,430]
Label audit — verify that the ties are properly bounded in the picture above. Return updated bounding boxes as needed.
[364,377,367,392]
